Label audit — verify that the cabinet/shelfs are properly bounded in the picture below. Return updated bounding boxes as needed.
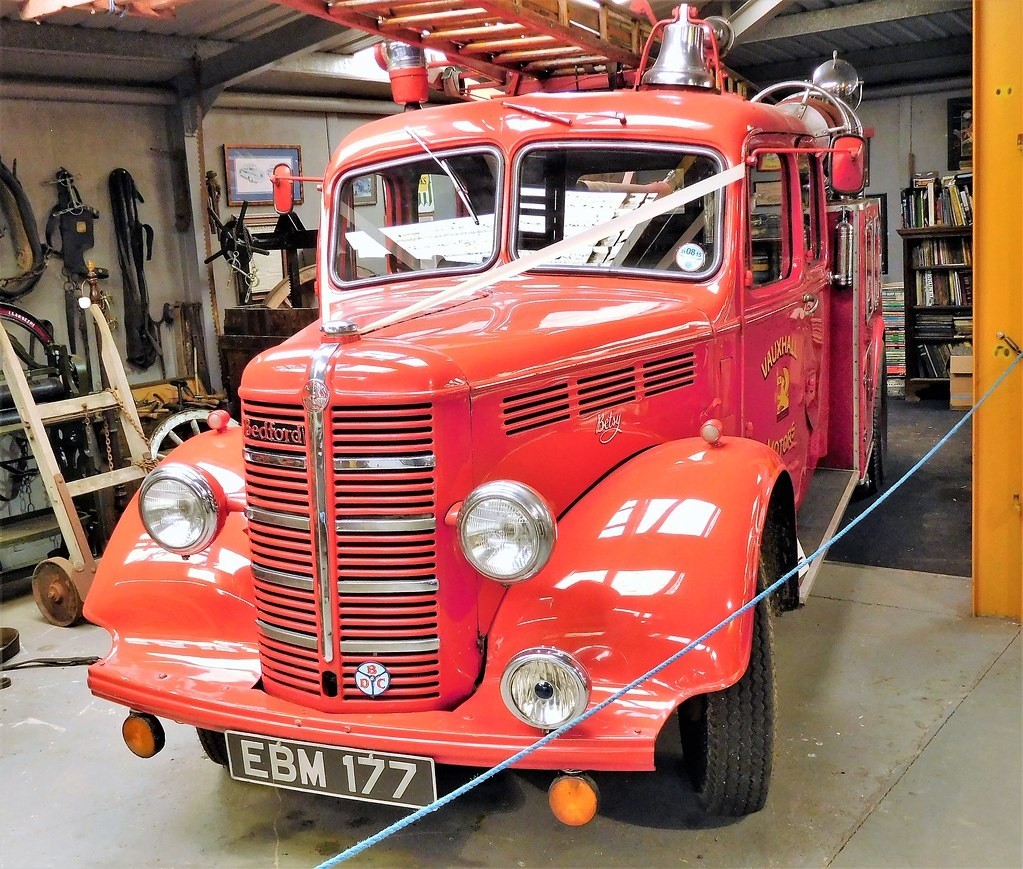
[896,188,973,401]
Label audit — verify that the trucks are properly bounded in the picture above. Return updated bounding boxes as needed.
[82,3,895,818]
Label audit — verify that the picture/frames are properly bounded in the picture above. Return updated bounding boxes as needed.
[865,193,889,275]
[223,142,306,206]
[229,214,290,305]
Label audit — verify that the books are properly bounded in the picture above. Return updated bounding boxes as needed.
[914,240,972,268]
[904,181,974,228]
[916,312,973,339]
[882,282,906,376]
[916,341,973,377]
[915,270,973,305]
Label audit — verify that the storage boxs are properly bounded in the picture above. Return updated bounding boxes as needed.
[943,345,974,411]
